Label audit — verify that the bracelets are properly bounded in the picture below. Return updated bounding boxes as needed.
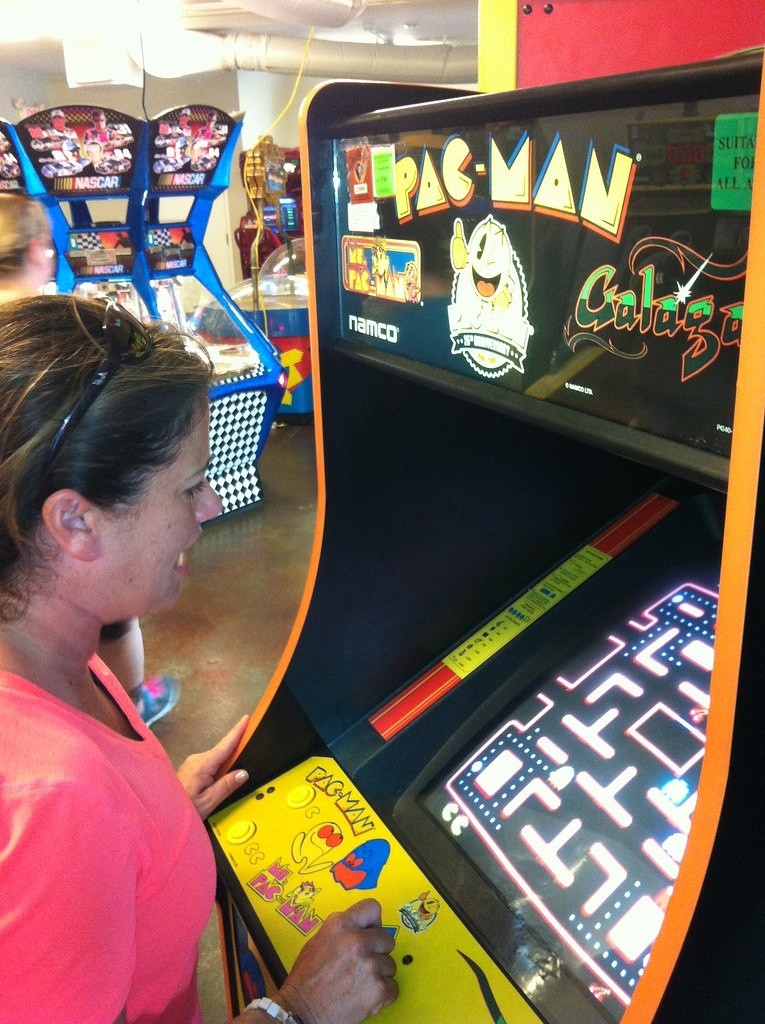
[241,997,298,1024]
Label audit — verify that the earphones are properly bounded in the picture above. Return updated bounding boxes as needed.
[60,509,90,531]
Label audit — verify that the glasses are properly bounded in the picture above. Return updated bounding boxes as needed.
[37,297,155,500]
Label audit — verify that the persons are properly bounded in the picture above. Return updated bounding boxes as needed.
[0,295,401,1024]
[0,193,177,726]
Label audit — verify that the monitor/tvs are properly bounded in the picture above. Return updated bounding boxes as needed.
[391,567,719,1024]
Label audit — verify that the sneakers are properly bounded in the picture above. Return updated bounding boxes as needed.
[128,672,180,728]
